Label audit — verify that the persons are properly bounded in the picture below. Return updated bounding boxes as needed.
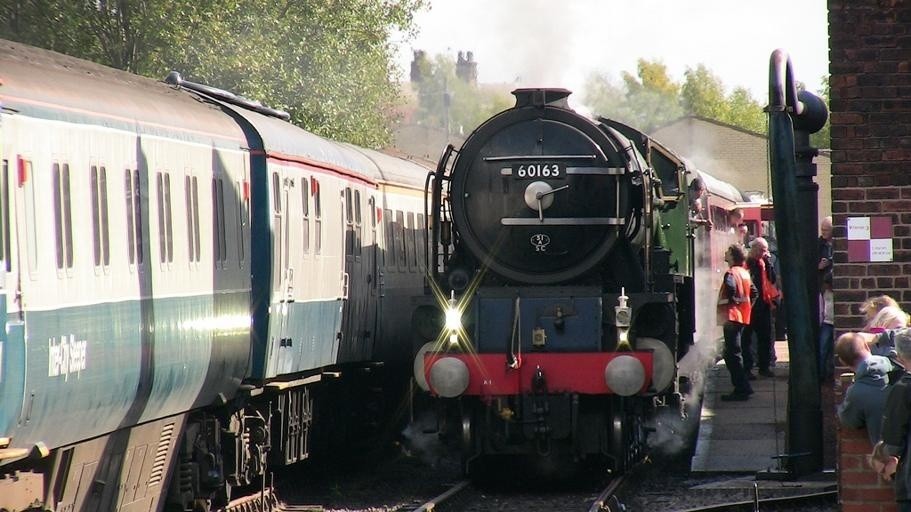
[716,208,833,401]
[835,295,911,512]
[687,178,714,232]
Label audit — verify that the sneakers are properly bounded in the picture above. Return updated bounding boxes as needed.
[720,388,754,401]
[744,367,775,379]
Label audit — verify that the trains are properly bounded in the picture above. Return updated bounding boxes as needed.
[0,38,450,512]
[406,83,776,489]
